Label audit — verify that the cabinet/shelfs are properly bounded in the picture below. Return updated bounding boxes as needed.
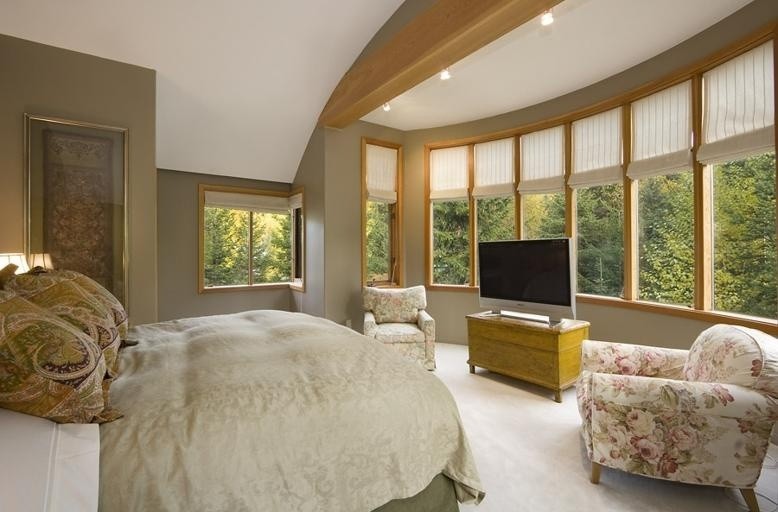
[465,311,590,403]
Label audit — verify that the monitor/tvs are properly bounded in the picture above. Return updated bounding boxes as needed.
[478,238,576,327]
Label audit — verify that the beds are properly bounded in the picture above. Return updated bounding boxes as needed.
[0,309,485,512]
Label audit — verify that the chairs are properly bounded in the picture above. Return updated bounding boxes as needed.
[363,285,435,371]
[576,323,778,512]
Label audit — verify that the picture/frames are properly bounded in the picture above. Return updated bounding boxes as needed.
[23,111,129,318]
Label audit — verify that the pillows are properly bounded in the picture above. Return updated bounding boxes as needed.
[0,264,139,423]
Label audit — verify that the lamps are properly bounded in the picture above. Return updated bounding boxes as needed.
[439,68,450,81]
[0,253,30,275]
[382,102,390,112]
[541,10,554,26]
[29,253,54,269]
